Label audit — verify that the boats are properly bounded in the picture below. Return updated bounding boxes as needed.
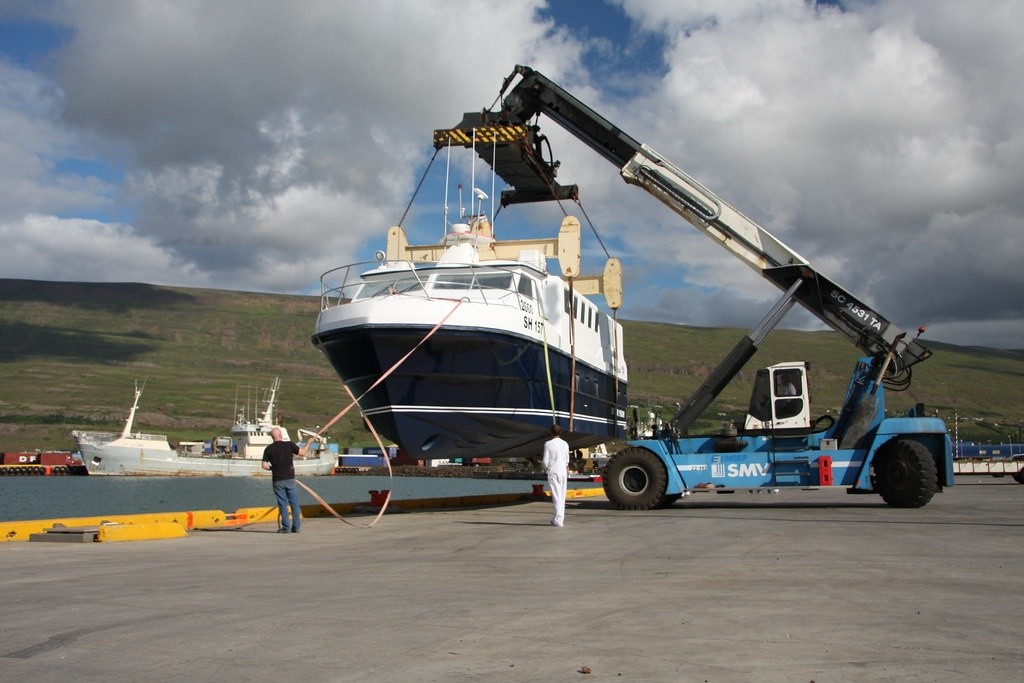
[70,376,335,476]
[311,128,629,464]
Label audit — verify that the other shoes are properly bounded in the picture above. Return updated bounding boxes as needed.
[278,529,291,532]
[550,521,558,526]
[291,528,300,532]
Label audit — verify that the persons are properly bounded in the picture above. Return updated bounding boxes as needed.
[541,424,569,527]
[778,373,796,405]
[212,437,220,455]
[261,428,316,533]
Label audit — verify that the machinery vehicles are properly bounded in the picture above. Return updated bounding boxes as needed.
[432,64,952,509]
